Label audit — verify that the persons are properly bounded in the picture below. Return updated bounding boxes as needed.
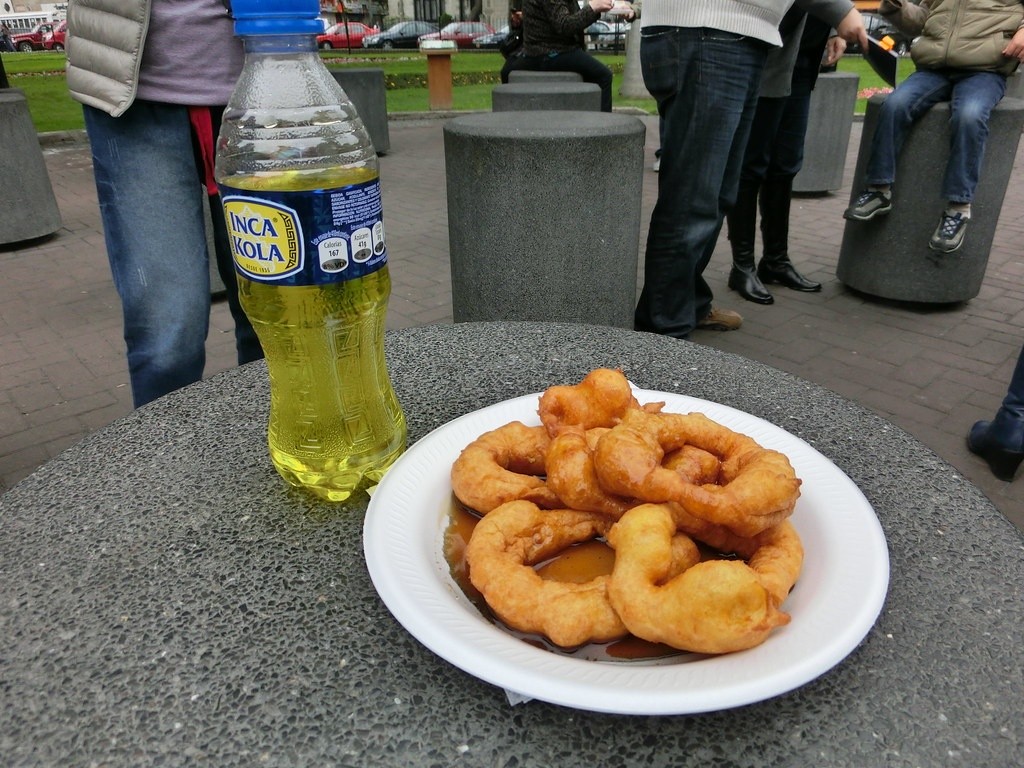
[619,0,869,340]
[65,0,268,411]
[43,28,53,40]
[843,0,1024,253]
[968,344,1024,482]
[500,0,613,112]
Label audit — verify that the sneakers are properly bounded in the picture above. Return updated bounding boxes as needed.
[696,303,743,331]
[843,189,892,222]
[928,208,971,253]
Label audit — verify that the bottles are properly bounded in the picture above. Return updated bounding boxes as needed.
[214,1,409,501]
[363,388,890,716]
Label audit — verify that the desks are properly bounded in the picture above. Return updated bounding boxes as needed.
[2,326,1024,768]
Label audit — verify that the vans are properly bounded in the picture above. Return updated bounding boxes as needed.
[41,19,67,53]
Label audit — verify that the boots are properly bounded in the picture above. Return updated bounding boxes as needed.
[968,349,1024,482]
[726,183,774,305]
[757,180,822,292]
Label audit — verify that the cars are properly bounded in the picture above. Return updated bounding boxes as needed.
[843,12,911,58]
[361,21,439,50]
[10,22,61,53]
[316,22,379,50]
[416,21,496,49]
[470,23,509,49]
[583,18,631,51]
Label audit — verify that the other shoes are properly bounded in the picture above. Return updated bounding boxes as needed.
[654,156,660,171]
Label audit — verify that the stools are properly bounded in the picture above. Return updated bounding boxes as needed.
[492,82,603,112]
[507,69,581,81]
[330,67,392,156]
[442,109,647,335]
[792,74,859,194]
[1,90,64,243]
[836,90,1022,307]
